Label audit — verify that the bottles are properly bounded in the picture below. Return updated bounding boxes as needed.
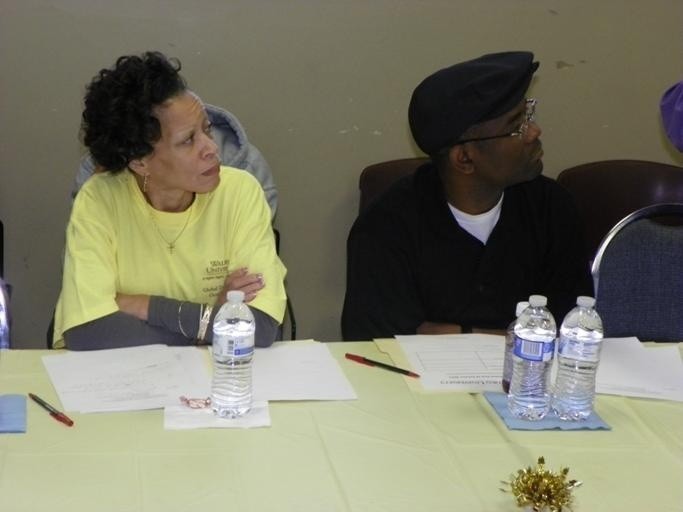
[506,294,556,421]
[501,301,530,394]
[210,290,256,416]
[551,294,605,421]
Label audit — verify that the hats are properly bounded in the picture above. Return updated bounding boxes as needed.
[409,51,540,157]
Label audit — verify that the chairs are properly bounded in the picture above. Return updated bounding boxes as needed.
[357,155,432,215]
[553,158,683,307]
[43,101,297,352]
[588,197,683,344]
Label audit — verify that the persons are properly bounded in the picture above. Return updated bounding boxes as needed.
[340,50,601,341]
[47,49,288,351]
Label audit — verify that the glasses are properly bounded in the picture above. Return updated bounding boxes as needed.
[451,99,538,148]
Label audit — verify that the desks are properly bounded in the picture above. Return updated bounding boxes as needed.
[0,334,682,512]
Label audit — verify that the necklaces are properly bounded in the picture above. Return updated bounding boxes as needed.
[133,176,192,255]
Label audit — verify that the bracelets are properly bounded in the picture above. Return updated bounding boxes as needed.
[178,301,214,340]
[461,325,473,334]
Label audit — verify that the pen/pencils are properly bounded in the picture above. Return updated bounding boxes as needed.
[29,393,74,426]
[345,353,420,379]
